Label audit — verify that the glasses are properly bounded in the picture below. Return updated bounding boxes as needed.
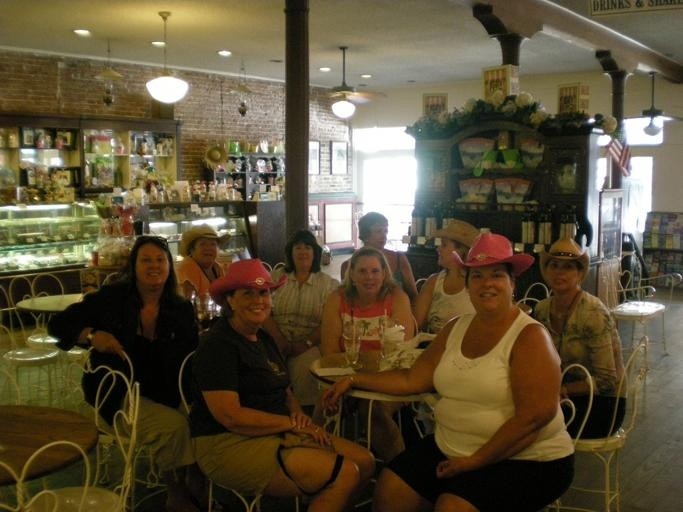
[324,402,340,435]
[133,234,167,249]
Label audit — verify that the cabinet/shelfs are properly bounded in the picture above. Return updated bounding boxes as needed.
[0,200,103,328]
[408,111,613,331]
[598,189,624,309]
[75,201,257,263]
[0,111,83,199]
[204,154,286,202]
[81,113,183,198]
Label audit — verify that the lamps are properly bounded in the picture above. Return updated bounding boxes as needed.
[145,12,190,104]
[95,40,124,106]
[230,60,253,117]
[642,71,665,135]
[331,47,356,119]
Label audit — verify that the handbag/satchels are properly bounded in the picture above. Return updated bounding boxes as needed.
[280,430,336,450]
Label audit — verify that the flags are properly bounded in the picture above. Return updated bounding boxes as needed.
[607,127,632,176]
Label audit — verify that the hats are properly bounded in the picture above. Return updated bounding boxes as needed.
[540,237,591,288]
[197,257,290,301]
[176,223,232,258]
[449,232,536,276]
[425,218,484,248]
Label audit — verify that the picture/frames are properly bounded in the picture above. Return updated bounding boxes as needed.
[324,202,353,244]
[308,204,319,237]
[330,141,348,176]
[308,140,320,176]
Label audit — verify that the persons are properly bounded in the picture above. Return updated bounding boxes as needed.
[322,232,576,512]
[533,238,626,441]
[48,212,479,470]
[184,258,376,512]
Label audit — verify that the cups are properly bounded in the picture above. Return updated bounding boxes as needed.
[343,317,406,371]
